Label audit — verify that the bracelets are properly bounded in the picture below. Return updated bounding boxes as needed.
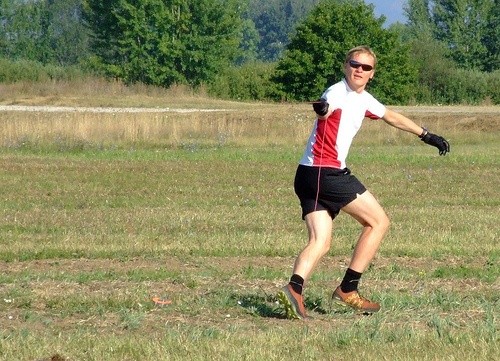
[418,127,427,138]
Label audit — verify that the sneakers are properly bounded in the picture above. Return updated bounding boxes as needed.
[332,286,380,312]
[279,284,308,320]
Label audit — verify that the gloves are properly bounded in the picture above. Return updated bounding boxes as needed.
[313,98,329,116]
[421,132,450,156]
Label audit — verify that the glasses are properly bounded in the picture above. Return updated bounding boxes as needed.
[348,60,374,71]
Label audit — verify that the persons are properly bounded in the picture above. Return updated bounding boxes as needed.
[279,45,450,319]
